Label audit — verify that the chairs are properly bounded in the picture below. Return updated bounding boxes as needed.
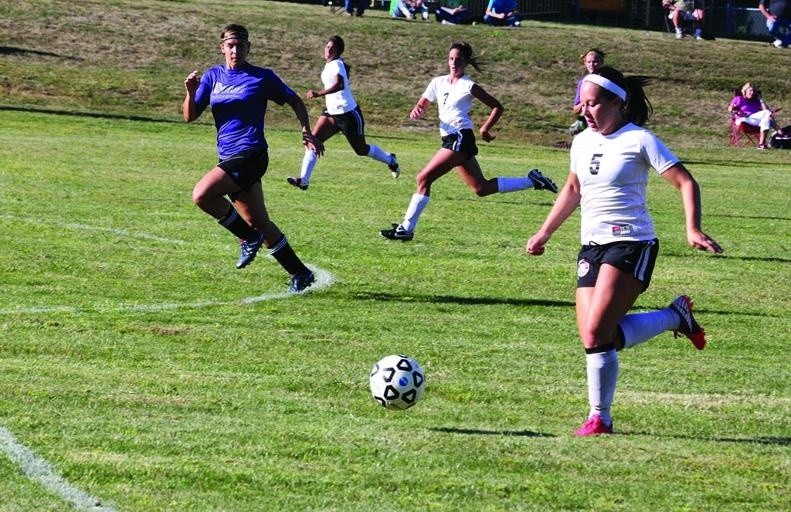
[665,8,695,36]
[730,113,761,148]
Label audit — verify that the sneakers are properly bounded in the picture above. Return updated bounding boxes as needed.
[575,415,611,436]
[289,270,314,292]
[669,295,705,350]
[235,232,264,268]
[758,143,767,149]
[527,169,557,192]
[387,153,399,177]
[379,224,413,241]
[286,176,308,190]
[776,134,790,139]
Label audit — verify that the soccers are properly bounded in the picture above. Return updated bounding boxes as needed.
[370,355,424,409]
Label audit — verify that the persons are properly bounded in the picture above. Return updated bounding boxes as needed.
[380,39,560,241]
[727,82,784,150]
[525,65,725,438]
[288,35,401,188]
[344,0,522,27]
[662,1,791,50]
[182,24,314,293]
